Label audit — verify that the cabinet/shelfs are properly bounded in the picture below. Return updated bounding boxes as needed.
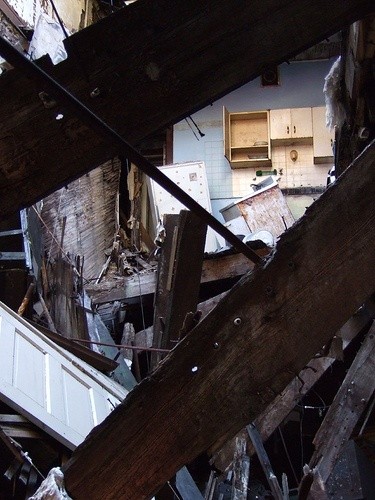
[221,103,336,165]
